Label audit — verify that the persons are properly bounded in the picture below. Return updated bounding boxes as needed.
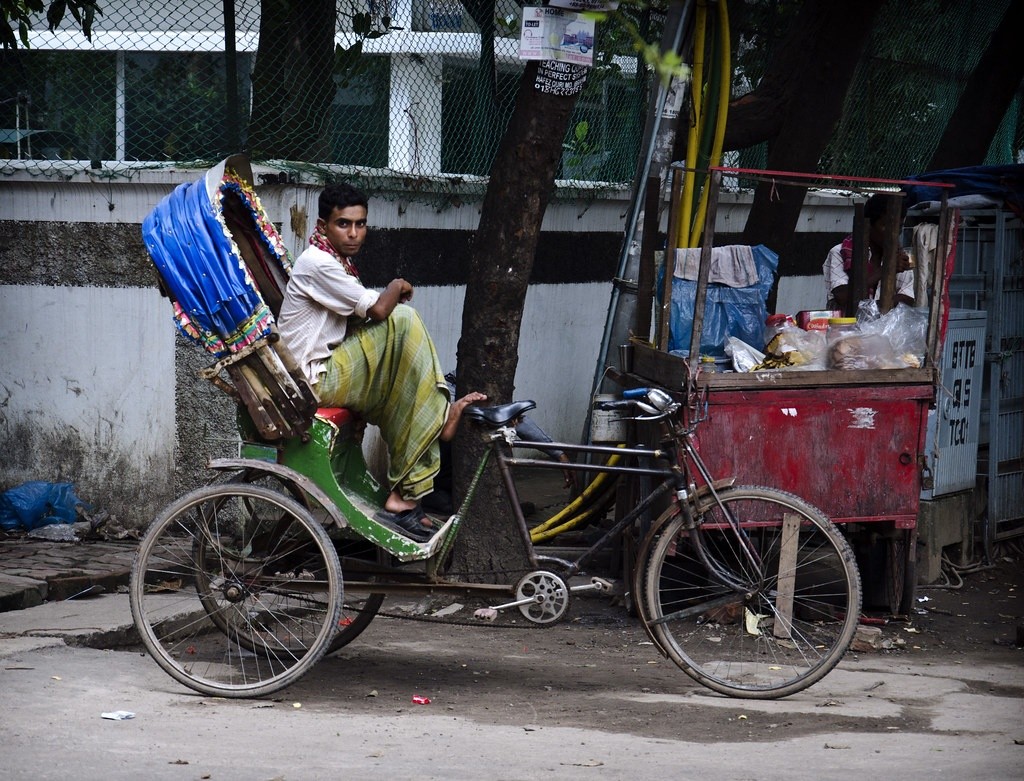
[268,183,489,542]
[823,192,915,318]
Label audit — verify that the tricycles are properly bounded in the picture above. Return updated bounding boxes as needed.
[127,152,863,700]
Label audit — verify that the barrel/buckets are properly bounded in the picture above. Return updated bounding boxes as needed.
[590,366,626,441]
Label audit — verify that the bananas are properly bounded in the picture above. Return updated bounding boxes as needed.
[752,354,787,372]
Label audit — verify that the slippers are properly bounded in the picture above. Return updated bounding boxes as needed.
[382,506,439,531]
[373,510,434,541]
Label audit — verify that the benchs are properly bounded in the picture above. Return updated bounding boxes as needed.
[311,408,355,482]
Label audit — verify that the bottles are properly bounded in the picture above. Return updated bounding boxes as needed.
[763,313,793,355]
[826,317,863,372]
